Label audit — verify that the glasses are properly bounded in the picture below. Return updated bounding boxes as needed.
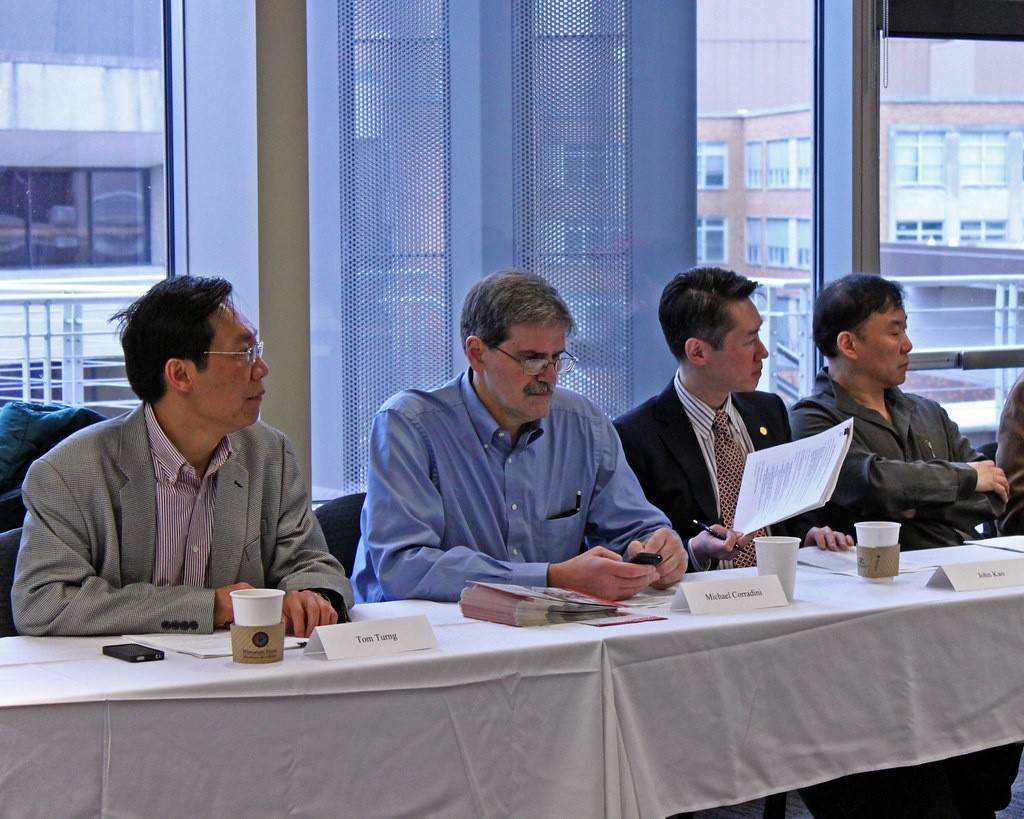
[202,340,265,364]
[480,341,579,376]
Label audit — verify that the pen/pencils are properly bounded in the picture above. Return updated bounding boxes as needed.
[693,519,747,554]
[576,490,581,513]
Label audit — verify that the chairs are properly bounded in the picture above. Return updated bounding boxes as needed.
[315,490,368,579]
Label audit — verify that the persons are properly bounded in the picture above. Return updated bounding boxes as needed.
[9,275,355,638]
[611,267,854,572]
[350,268,688,604]
[995,369,1024,536]
[788,272,1010,551]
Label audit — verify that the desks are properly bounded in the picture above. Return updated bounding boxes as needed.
[514,537,1024,819]
[0,598,614,818]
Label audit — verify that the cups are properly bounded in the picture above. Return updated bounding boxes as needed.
[854,522,902,584]
[752,536,801,602]
[230,589,286,626]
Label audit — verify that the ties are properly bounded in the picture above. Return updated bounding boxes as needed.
[709,408,767,568]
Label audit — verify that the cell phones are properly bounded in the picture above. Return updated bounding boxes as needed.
[102,643,165,663]
[630,552,663,566]
